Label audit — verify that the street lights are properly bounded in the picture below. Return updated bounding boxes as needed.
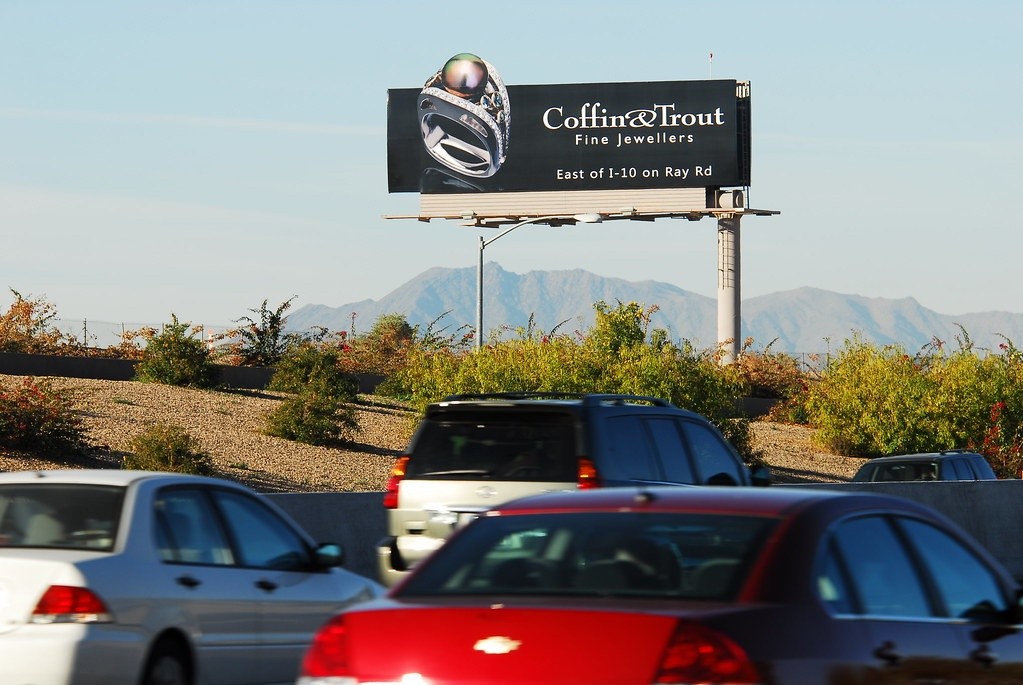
[475,212,603,345]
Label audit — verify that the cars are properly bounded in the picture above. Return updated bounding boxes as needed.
[0,470,392,684]
[295,485,1023,685]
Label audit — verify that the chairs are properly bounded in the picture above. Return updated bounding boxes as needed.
[486,557,640,589]
[692,557,744,598]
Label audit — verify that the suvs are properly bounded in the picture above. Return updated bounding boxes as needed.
[377,390,771,594]
[850,448,996,483]
